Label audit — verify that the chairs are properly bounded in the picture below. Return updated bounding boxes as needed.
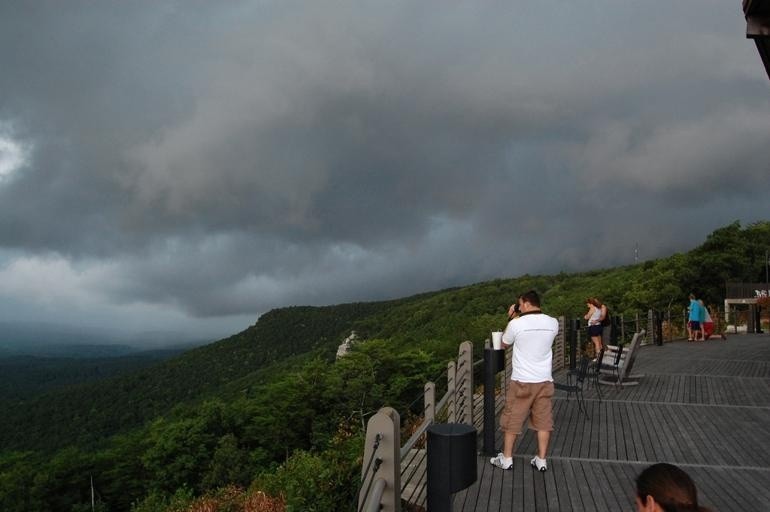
[550,329,646,421]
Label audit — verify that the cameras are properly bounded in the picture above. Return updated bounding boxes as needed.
[514,303,521,313]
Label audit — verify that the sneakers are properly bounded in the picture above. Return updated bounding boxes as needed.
[530,456,548,472]
[489,453,514,470]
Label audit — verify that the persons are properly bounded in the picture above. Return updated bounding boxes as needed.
[488,290,559,472]
[633,463,710,512]
[688,294,700,341]
[686,298,727,340]
[591,296,612,351]
[584,298,602,362]
[686,300,705,341]
